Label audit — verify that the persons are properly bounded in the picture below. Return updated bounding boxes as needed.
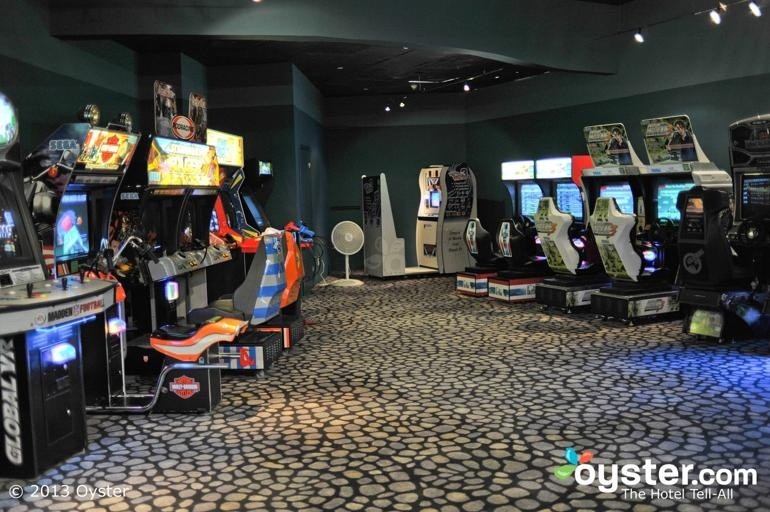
[666,120,699,161]
[605,127,634,166]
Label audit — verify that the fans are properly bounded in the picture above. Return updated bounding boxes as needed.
[331,220,365,287]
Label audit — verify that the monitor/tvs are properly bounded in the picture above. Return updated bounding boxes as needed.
[0,185,22,258]
[55,194,90,257]
[599,183,635,214]
[520,183,544,218]
[556,183,583,220]
[242,195,265,228]
[742,178,770,218]
[502,182,516,217]
[657,182,696,220]
[209,206,220,232]
[179,208,193,247]
[686,197,704,213]
[431,192,441,207]
